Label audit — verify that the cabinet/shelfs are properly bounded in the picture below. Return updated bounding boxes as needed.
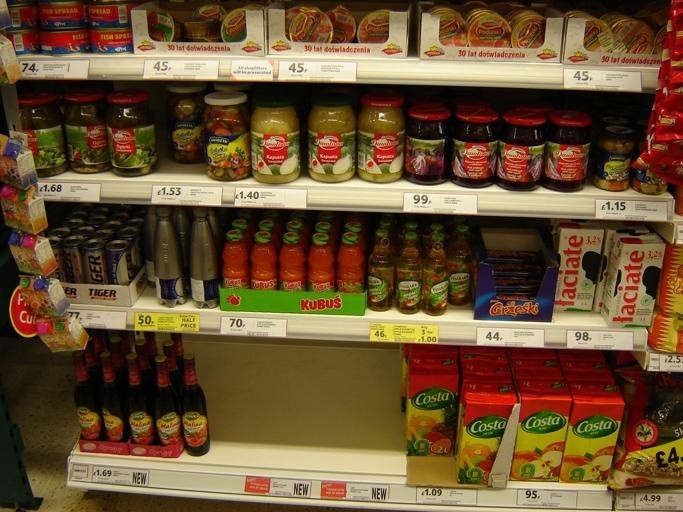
[0,42,682,511]
[608,211,680,511]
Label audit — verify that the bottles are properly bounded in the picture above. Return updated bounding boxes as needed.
[222,207,365,296]
[366,216,473,317]
[70,330,211,459]
[144,203,220,311]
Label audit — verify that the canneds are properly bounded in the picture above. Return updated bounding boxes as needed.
[44,203,148,286]
[0,0,145,55]
[19,82,669,195]
